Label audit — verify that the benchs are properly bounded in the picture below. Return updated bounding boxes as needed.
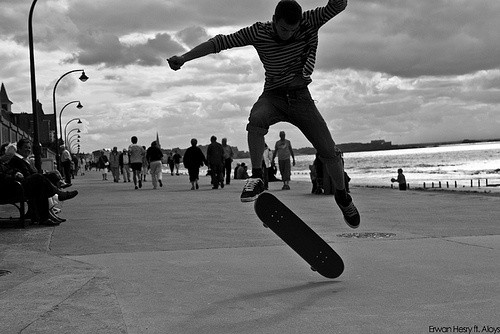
[0,163,28,222]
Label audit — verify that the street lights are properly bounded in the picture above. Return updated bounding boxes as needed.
[70,145,80,150]
[71,138,80,143]
[72,148,80,151]
[67,128,81,145]
[53,69,89,139]
[69,134,80,143]
[60,100,83,139]
[65,118,82,146]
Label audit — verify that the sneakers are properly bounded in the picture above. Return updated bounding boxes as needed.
[334,194,360,228]
[52,207,61,212]
[287,184,290,189]
[282,185,286,189]
[241,175,267,202]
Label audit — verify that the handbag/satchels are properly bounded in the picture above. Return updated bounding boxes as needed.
[104,161,110,165]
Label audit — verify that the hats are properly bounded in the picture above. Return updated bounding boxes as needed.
[279,130,285,135]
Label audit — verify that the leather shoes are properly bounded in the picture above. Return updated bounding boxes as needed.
[58,190,78,201]
[49,210,62,222]
[39,219,60,225]
[61,183,72,188]
[51,208,66,222]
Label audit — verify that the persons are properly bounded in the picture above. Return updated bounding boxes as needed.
[205,136,224,189]
[269,130,296,190]
[57,144,73,184]
[127,136,145,190]
[78,155,87,176]
[234,162,249,179]
[220,137,235,185]
[182,137,208,189]
[109,146,121,182]
[164,0,363,228]
[17,139,41,175]
[145,141,164,189]
[166,153,175,175]
[119,148,131,183]
[1,142,78,225]
[173,150,182,175]
[98,150,110,181]
[390,167,408,190]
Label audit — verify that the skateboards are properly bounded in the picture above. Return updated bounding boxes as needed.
[254,192,344,279]
